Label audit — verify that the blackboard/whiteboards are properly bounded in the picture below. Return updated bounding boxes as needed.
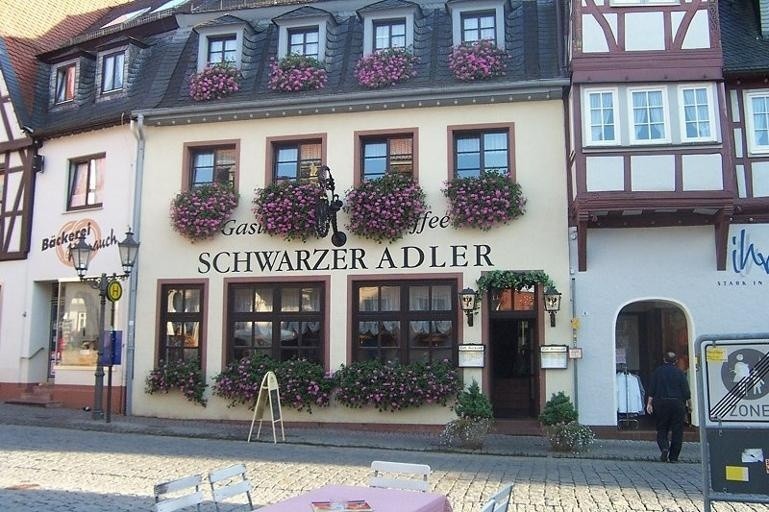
[268,387,282,423]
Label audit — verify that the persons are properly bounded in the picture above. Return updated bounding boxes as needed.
[646,351,692,462]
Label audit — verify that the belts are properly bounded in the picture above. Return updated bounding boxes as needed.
[656,397,680,401]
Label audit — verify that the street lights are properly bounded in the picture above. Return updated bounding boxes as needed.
[68,226,142,421]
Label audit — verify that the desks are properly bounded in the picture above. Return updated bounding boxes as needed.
[247,482,454,512]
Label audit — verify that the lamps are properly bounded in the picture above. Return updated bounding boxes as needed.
[457,286,480,327]
[539,284,562,327]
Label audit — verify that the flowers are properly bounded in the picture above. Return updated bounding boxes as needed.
[447,37,512,83]
[266,47,326,92]
[172,179,240,242]
[542,418,603,455]
[352,43,422,88]
[342,170,431,242]
[252,177,329,241]
[436,416,498,449]
[442,168,528,231]
[144,356,206,409]
[210,355,330,411]
[188,55,245,101]
[331,359,458,407]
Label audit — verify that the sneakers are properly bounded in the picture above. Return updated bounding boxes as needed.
[660,448,678,463]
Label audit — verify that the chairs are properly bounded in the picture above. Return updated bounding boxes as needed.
[368,461,431,494]
[489,483,514,512]
[479,495,499,511]
[208,461,255,512]
[154,472,204,511]
